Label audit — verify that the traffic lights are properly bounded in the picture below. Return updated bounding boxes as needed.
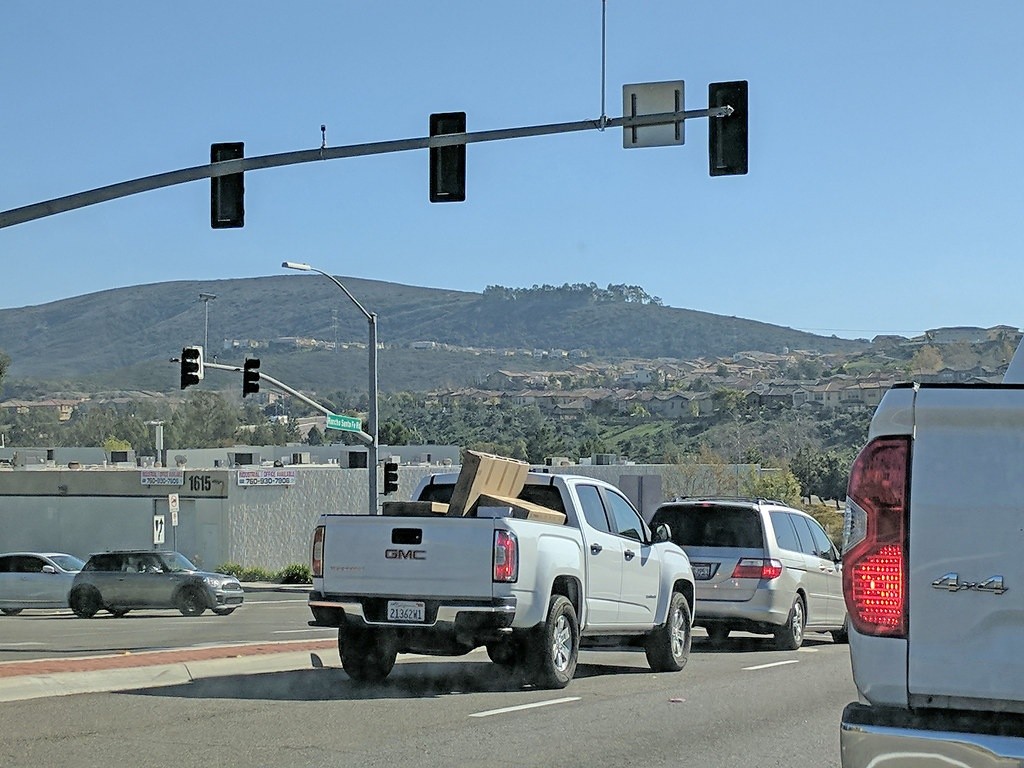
[243,356,263,399]
[182,348,200,390]
[383,462,400,496]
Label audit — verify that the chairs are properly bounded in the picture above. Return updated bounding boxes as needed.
[125,557,137,572]
[713,530,739,548]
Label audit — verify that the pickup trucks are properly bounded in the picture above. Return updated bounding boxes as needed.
[838,332,1023,768]
[306,472,699,690]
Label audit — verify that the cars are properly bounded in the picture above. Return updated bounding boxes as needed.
[69,550,244,617]
[649,497,850,652]
[0,553,87,617]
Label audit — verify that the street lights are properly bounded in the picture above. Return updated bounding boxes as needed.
[282,261,379,515]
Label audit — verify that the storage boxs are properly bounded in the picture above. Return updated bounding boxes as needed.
[382,450,566,525]
